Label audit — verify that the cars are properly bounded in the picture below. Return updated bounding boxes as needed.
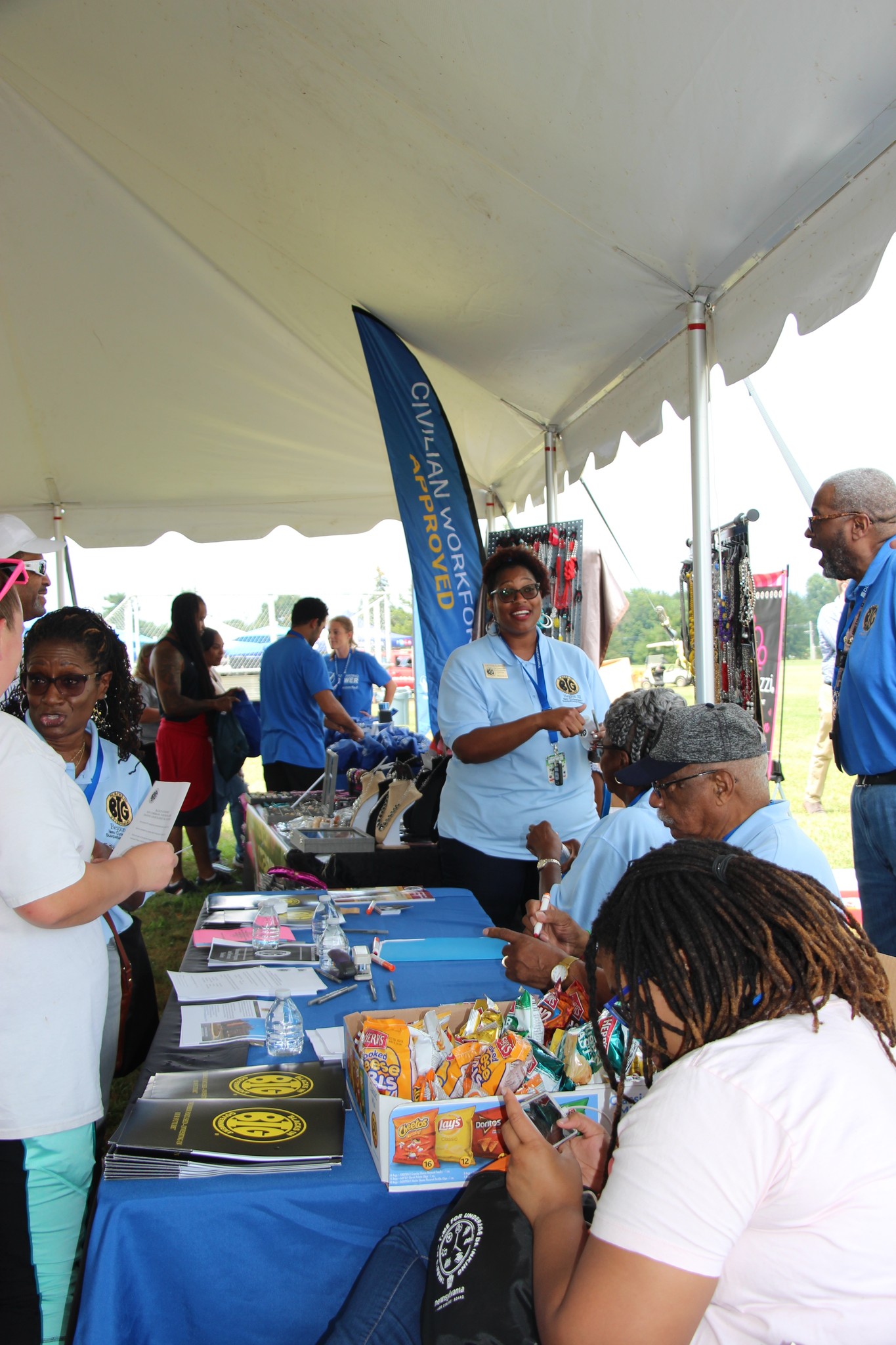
[640,663,692,690]
[353,627,416,686]
[114,628,157,661]
[225,634,288,656]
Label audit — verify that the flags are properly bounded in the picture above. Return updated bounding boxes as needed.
[351,304,488,738]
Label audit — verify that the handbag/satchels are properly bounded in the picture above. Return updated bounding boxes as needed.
[114,915,160,1079]
[210,687,262,782]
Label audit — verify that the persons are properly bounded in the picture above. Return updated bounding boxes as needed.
[128,591,240,896]
[484,702,843,1010]
[798,468,895,1030]
[5,606,152,1132]
[321,615,396,736]
[316,838,896,1345]
[526,686,688,932]
[199,625,251,864]
[432,546,612,941]
[130,643,162,785]
[257,597,364,793]
[0,553,179,1345]
[1,512,66,671]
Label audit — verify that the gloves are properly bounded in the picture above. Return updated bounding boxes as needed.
[341,731,351,739]
[359,731,387,758]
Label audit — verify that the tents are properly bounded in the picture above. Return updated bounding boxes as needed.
[0,0,896,704]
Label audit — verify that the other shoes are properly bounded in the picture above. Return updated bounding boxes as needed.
[213,852,244,868]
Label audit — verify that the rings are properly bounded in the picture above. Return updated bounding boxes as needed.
[502,955,509,968]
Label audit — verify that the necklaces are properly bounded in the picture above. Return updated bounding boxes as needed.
[67,738,85,770]
[496,526,583,644]
[679,545,756,718]
[352,755,444,832]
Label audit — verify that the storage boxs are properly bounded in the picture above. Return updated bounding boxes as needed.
[341,1000,612,1192]
[291,827,375,853]
[264,748,339,824]
[245,792,294,804]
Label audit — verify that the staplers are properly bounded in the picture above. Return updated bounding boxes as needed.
[351,945,373,982]
[326,948,356,978]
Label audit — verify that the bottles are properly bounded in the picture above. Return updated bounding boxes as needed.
[318,917,350,973]
[312,895,338,944]
[251,900,280,954]
[265,988,304,1056]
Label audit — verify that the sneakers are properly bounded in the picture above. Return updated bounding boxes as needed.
[196,870,241,887]
[165,877,202,897]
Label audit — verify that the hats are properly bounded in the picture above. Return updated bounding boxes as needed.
[614,702,767,787]
[0,513,67,559]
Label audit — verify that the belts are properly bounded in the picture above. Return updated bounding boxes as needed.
[854,770,896,787]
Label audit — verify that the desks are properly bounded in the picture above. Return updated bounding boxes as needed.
[237,788,442,893]
[77,886,543,1345]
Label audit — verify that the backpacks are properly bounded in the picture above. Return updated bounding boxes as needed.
[420,1153,598,1345]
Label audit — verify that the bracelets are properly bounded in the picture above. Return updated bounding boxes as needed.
[585,929,591,937]
[346,768,367,784]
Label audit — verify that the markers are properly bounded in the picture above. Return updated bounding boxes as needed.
[533,892,553,938]
[370,953,395,971]
[366,900,376,914]
[372,936,379,956]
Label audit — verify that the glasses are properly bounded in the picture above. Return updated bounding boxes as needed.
[603,973,645,1029]
[20,671,104,697]
[595,742,632,763]
[2,559,47,576]
[807,512,872,534]
[651,770,737,799]
[491,582,541,603]
[0,559,29,601]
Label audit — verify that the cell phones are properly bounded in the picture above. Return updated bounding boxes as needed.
[520,1091,579,1149]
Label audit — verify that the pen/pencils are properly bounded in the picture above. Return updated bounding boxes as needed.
[307,983,359,1006]
[389,980,396,1000]
[342,929,388,935]
[315,967,342,984]
[369,979,377,1000]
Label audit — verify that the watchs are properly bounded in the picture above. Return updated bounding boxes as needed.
[537,858,562,875]
[551,955,580,988]
